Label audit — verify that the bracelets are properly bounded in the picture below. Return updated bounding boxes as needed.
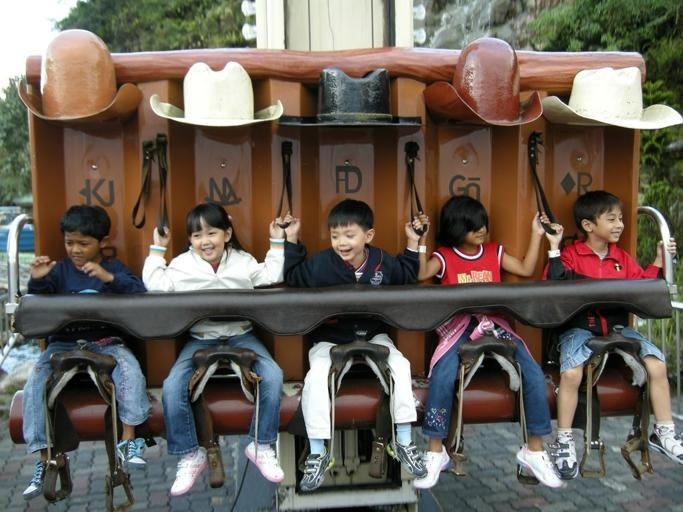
[268,237,288,246]
[146,245,168,254]
[547,249,561,258]
[417,246,426,254]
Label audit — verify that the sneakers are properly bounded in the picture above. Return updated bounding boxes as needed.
[649,424,682,464]
[23,459,51,497]
[245,442,285,484]
[388,439,427,479]
[516,444,565,489]
[117,438,148,472]
[170,449,207,497]
[413,446,450,489]
[300,445,335,491]
[553,436,578,479]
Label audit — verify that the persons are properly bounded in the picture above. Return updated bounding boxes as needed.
[546,190,683,478]
[22,205,150,498]
[413,195,563,489]
[282,198,428,491]
[141,202,285,495]
